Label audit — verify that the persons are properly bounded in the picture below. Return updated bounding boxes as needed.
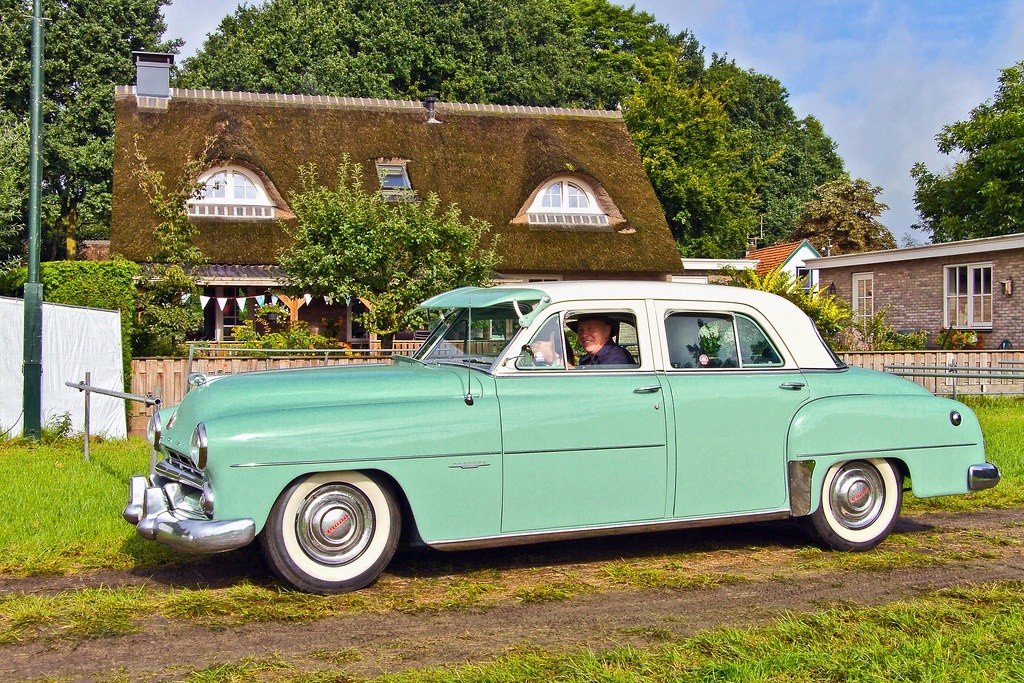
[530,311,638,366]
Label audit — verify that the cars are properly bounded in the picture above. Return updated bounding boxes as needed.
[124,281,999,595]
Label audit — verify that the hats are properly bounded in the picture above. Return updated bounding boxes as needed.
[566,313,619,337]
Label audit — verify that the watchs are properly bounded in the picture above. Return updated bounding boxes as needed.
[556,354,562,366]
